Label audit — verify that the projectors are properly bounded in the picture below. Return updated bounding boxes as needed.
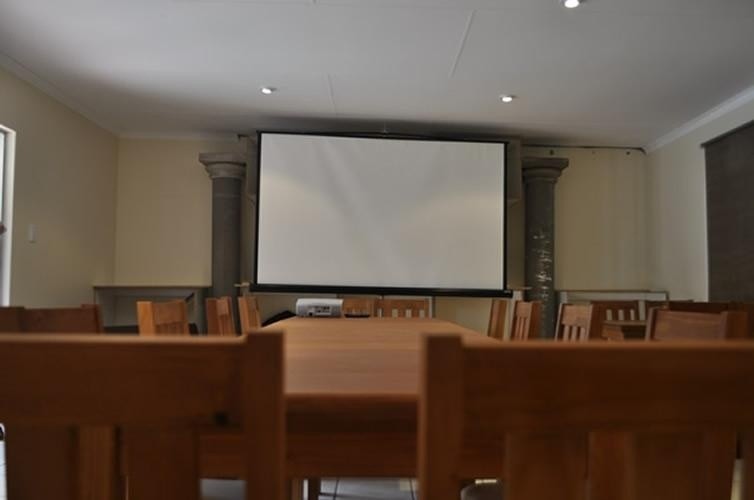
[296,297,344,317]
